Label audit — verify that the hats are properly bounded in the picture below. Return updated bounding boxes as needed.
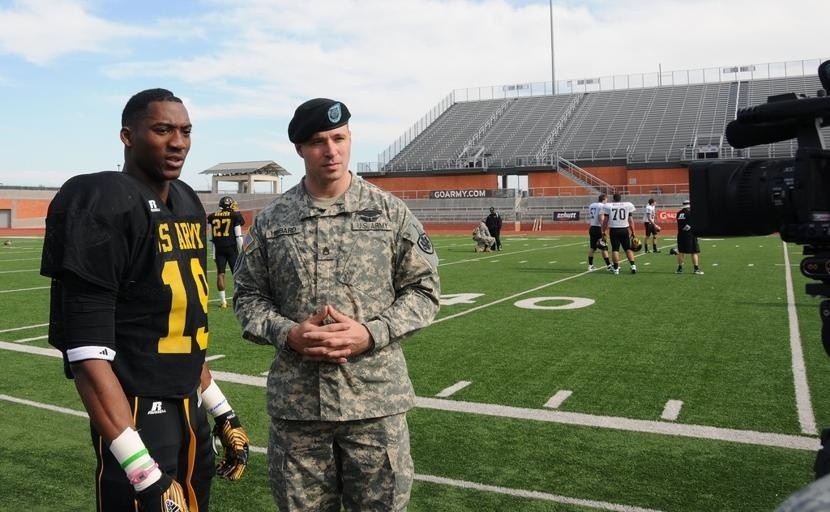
[681,200,691,208]
[288,99,351,143]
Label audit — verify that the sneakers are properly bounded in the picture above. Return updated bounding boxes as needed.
[645,251,650,253]
[630,265,636,273]
[653,250,660,253]
[607,264,621,275]
[587,265,597,270]
[673,270,683,274]
[694,269,704,274]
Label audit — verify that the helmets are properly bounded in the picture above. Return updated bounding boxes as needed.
[670,248,679,255]
[219,196,240,211]
[630,236,642,252]
[596,238,608,250]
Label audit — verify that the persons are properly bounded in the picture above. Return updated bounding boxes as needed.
[674,200,706,276]
[485,206,503,252]
[641,198,662,255]
[600,193,638,275]
[584,194,622,272]
[228,95,442,512]
[40,86,252,512]
[472,219,495,253]
[207,195,246,309]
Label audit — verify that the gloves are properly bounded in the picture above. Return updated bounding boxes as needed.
[211,413,249,481]
[132,468,190,512]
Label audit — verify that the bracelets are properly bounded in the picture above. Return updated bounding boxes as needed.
[126,458,155,481]
[120,448,149,471]
[129,462,159,486]
